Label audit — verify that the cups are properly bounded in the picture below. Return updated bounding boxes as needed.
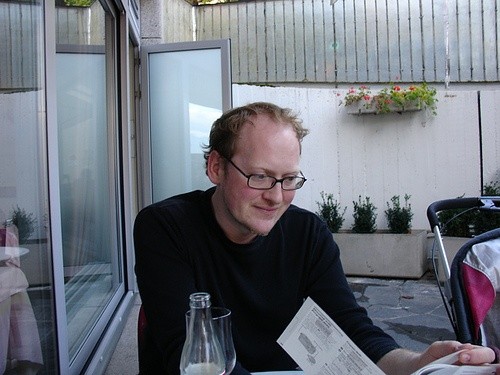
[184,306,237,375]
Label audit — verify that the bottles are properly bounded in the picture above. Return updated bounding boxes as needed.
[179,292,227,375]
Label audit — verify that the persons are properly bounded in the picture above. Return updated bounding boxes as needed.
[134,102,497,375]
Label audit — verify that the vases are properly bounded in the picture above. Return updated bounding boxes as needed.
[346,94,422,115]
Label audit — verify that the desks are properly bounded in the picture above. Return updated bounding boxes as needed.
[1,242,31,267]
[0,266,45,374]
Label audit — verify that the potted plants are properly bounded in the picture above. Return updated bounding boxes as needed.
[313,191,429,279]
[436,181,500,281]
[3,205,52,285]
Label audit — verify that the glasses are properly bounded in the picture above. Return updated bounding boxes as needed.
[220,153,307,191]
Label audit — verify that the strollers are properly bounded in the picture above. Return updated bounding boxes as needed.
[427,196,500,365]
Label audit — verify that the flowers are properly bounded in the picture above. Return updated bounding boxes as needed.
[336,81,442,128]
